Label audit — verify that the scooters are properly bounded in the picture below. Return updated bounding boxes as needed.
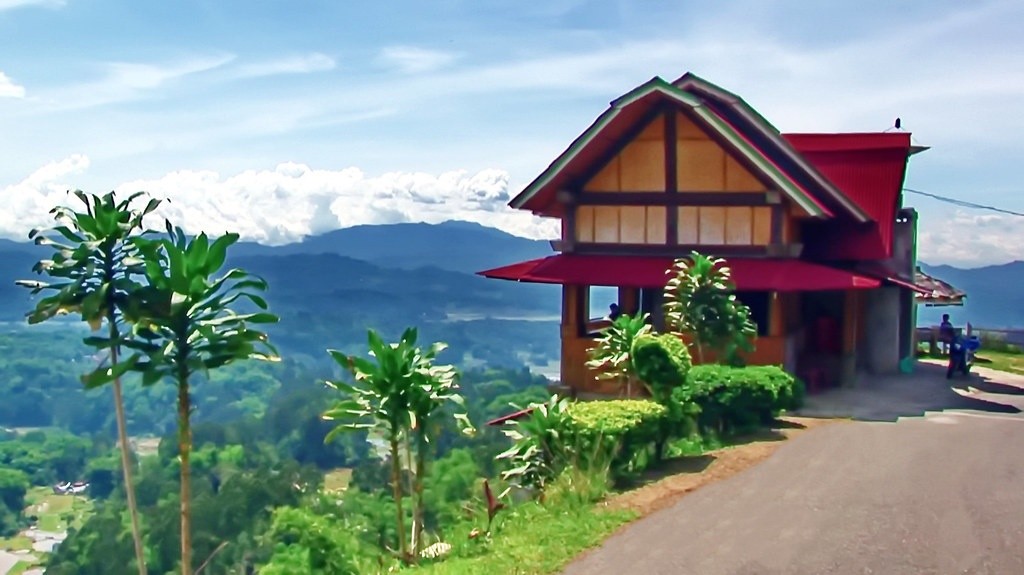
[945,334,980,379]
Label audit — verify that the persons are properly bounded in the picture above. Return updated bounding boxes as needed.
[940,314,954,354]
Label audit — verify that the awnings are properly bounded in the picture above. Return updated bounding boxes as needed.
[853,263,968,304]
